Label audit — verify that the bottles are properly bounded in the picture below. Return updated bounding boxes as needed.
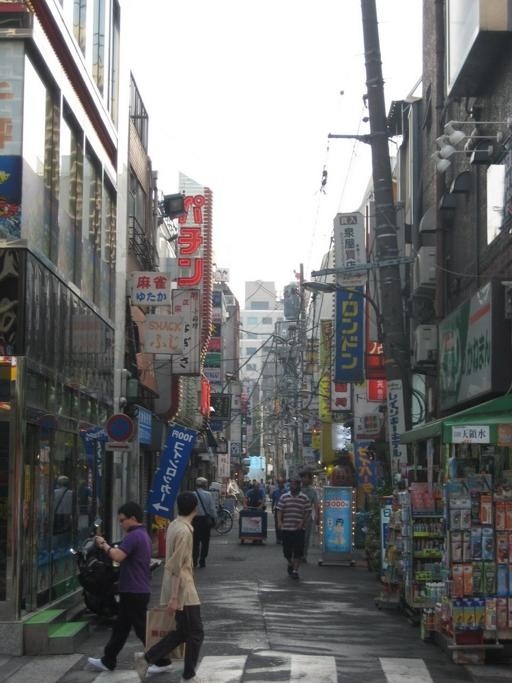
[409,515,449,603]
[381,499,393,569]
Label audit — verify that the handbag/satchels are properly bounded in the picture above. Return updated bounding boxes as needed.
[78,555,111,595]
[142,606,187,661]
[207,513,215,527]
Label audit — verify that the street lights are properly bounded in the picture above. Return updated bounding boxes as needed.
[297,276,385,351]
[252,377,266,479]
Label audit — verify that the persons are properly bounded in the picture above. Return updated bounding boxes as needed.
[87,501,173,675]
[192,469,321,580]
[52,475,74,551]
[133,490,205,683]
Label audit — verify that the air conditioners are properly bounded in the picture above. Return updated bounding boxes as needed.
[408,244,440,291]
[408,322,438,369]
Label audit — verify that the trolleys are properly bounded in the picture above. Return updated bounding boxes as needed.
[236,505,270,544]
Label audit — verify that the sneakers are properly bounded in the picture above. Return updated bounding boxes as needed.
[146,660,172,674]
[287,564,293,575]
[179,672,212,683]
[291,567,299,580]
[133,650,152,682]
[86,656,113,671]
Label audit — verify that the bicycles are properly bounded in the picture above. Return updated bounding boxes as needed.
[211,497,236,535]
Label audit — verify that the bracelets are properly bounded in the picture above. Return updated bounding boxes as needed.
[100,540,108,545]
[107,547,113,556]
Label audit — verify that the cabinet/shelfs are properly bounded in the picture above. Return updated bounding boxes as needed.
[401,493,446,615]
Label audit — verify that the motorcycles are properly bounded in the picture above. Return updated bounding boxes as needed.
[67,516,146,622]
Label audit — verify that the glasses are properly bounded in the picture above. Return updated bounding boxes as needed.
[118,517,127,523]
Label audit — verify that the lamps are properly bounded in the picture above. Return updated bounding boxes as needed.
[436,129,504,159]
[431,142,493,172]
[445,116,512,146]
